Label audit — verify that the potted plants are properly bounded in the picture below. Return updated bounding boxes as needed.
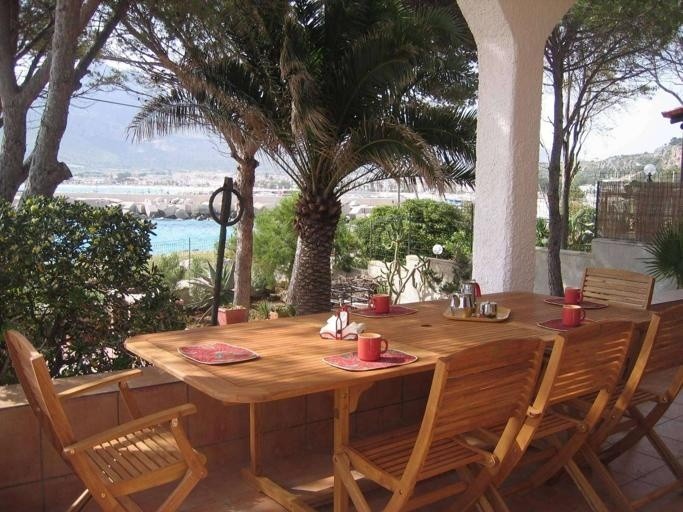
[186,260,249,325]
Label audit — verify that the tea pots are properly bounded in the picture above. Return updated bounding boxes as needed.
[450,293,471,317]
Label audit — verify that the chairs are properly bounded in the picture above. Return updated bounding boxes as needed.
[3,329,209,511]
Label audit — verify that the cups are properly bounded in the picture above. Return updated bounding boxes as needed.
[357,333,388,362]
[459,280,477,312]
[564,286,584,305]
[368,293,390,314]
[561,304,585,327]
[480,301,497,317]
